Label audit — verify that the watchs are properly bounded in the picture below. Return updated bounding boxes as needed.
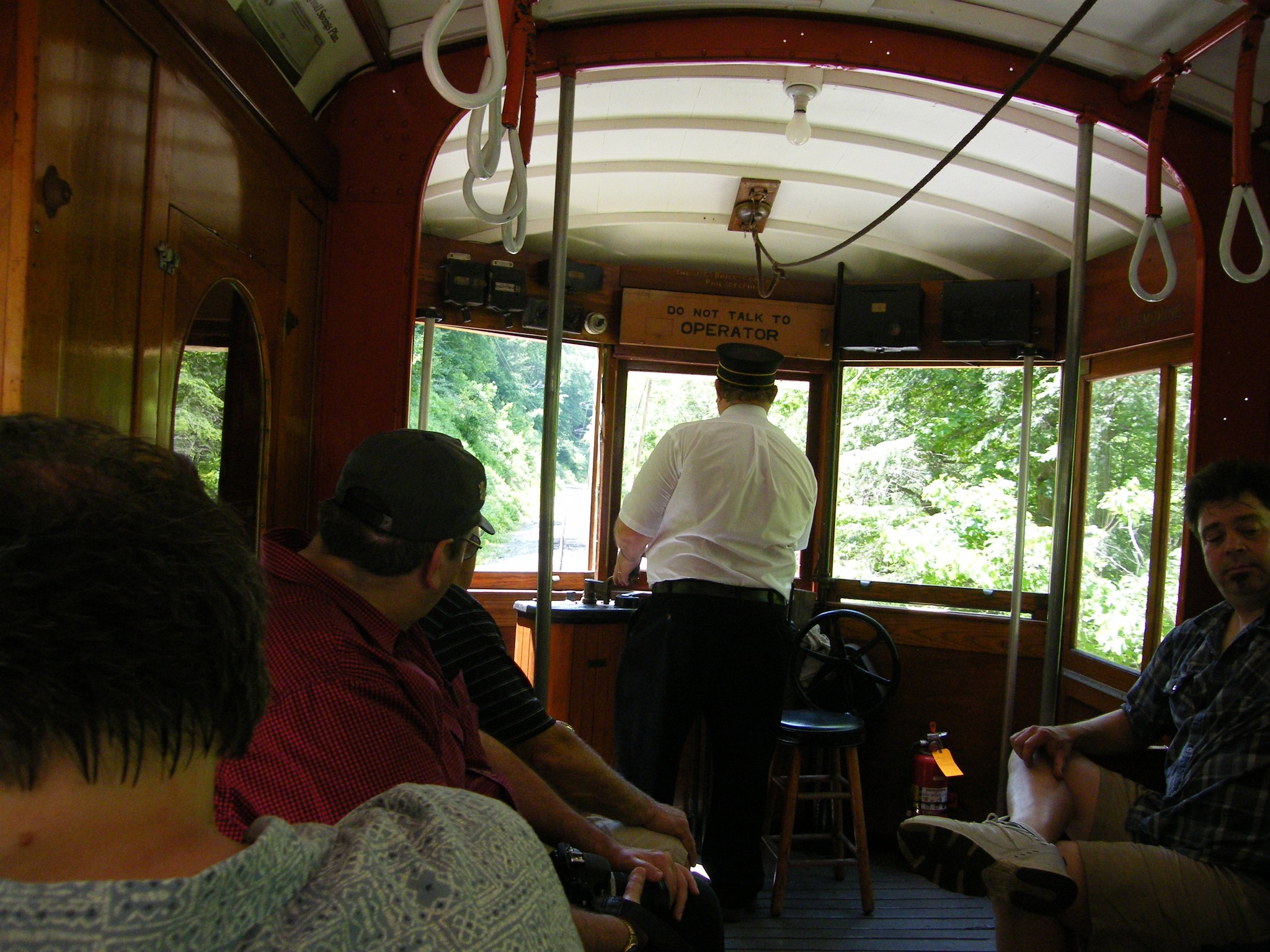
[618,918,639,951]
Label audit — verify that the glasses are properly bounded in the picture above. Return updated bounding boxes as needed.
[447,532,483,560]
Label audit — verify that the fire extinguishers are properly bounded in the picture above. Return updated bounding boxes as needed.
[904,720,964,816]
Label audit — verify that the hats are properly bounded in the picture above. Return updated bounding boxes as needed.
[715,343,785,392]
[333,429,486,542]
[394,428,496,535]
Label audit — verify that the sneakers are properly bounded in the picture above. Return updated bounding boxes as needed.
[897,812,1079,918]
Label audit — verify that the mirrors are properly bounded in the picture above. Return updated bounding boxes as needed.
[179,278,267,566]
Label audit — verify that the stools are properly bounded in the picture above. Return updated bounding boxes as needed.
[754,709,878,917]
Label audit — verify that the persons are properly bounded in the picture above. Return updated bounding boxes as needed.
[0,414,592,952]
[216,427,724,952]
[898,458,1270,952]
[605,340,818,920]
[413,516,702,871]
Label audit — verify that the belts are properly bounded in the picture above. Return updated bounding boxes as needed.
[652,580,789,608]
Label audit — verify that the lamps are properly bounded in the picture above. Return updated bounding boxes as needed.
[778,66,827,147]
